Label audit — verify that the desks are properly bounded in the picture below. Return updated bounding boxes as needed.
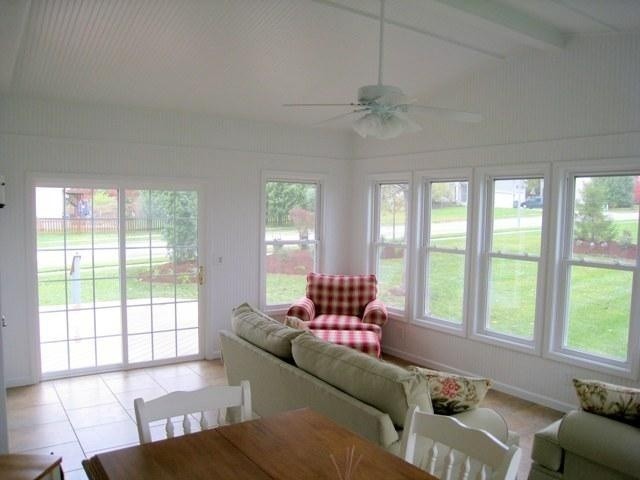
[81,405,438,480]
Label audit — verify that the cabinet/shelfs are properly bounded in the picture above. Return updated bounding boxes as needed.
[0,454,61,480]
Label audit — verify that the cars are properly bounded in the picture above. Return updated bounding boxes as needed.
[521,197,543,208]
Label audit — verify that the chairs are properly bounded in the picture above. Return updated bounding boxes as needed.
[526,409,639,479]
[134,379,252,445]
[286,272,389,359]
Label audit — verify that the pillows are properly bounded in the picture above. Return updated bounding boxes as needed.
[403,364,491,415]
[572,376,640,427]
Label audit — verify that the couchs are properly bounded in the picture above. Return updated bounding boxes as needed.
[219,301,520,480]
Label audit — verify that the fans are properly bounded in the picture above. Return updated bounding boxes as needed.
[278,0,486,141]
